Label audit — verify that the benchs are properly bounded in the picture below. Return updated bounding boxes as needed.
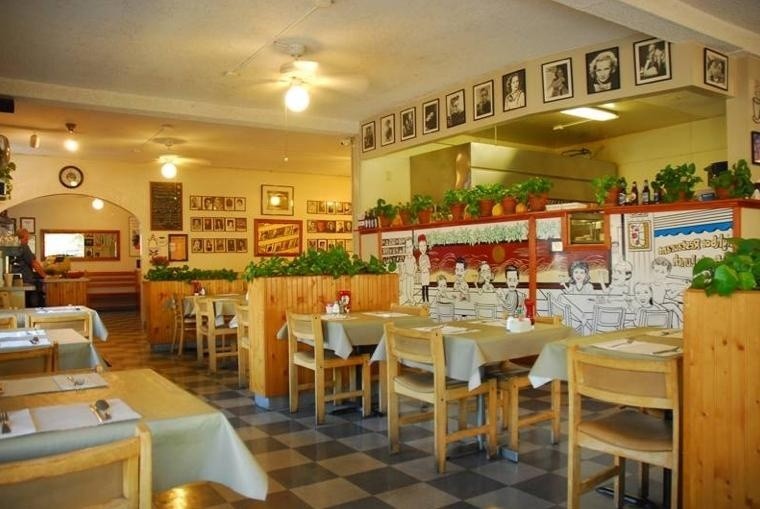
[79,271,141,316]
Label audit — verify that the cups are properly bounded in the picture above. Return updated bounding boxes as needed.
[13,279,24,287]
[3,273,13,286]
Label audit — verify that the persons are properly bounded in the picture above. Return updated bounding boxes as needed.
[11,228,47,307]
[308,201,352,252]
[708,58,725,83]
[191,197,247,253]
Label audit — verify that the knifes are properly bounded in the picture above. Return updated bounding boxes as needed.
[88,403,102,424]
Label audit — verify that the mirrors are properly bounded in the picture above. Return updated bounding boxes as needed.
[40,229,121,263]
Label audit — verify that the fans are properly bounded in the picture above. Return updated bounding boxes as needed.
[225,36,371,113]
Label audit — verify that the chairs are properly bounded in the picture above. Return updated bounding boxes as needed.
[170,293,249,389]
[286,301,574,475]
[565,325,683,509]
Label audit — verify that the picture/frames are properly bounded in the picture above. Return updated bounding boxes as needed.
[445,89,466,128]
[633,38,672,87]
[400,106,416,139]
[502,67,527,113]
[472,79,494,121]
[7,217,16,237]
[168,196,249,261]
[751,130,760,165]
[585,46,621,95]
[363,120,376,153]
[541,58,575,104]
[380,113,396,146]
[253,184,353,258]
[704,46,729,91]
[20,218,37,235]
[420,98,439,135]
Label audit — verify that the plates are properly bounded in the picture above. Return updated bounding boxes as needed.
[52,372,109,391]
[1,408,36,438]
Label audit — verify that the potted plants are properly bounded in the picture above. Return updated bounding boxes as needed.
[593,160,754,207]
[376,176,548,229]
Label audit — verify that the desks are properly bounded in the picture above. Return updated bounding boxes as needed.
[1,305,221,508]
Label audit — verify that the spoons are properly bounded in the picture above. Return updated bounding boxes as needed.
[94,399,110,420]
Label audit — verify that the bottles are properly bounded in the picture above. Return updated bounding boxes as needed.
[192,286,206,296]
[325,300,344,316]
[364,209,378,228]
[618,180,662,205]
[505,304,532,331]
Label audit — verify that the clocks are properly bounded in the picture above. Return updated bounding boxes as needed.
[60,166,83,189]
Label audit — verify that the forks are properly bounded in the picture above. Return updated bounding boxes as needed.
[1,412,11,433]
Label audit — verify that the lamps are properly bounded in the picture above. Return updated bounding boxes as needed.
[284,76,311,112]
